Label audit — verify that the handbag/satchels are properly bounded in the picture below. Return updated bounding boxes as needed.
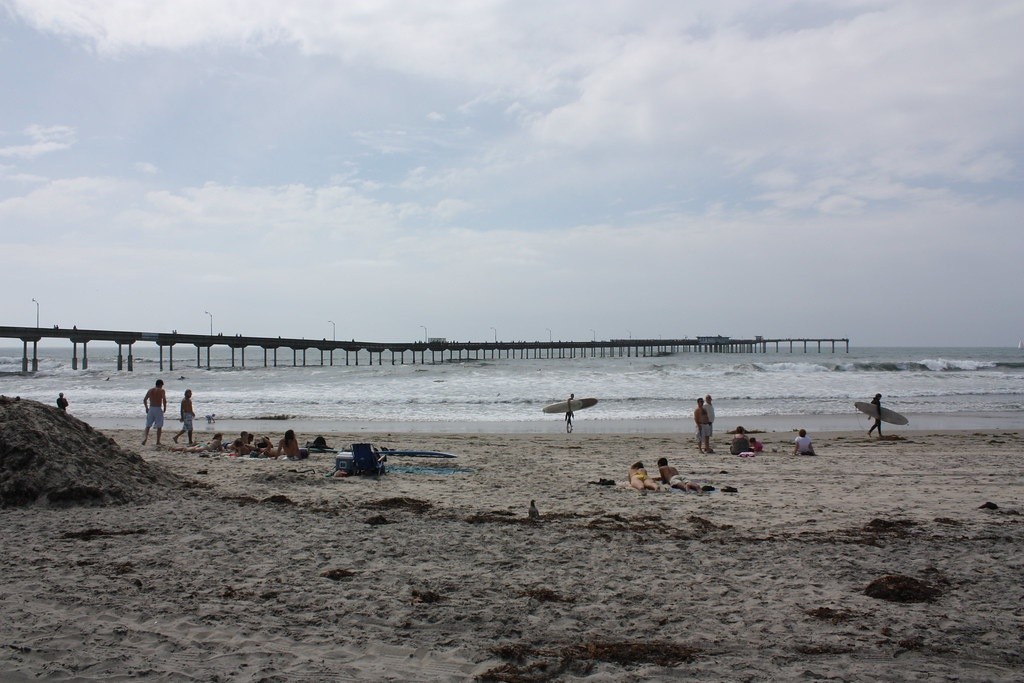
[738,451,756,458]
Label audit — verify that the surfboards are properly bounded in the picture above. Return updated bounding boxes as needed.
[542,397,599,414]
[854,401,909,426]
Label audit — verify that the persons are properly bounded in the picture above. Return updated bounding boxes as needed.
[173,430,334,461]
[694,395,763,455]
[141,379,166,446]
[657,457,702,494]
[867,393,883,437]
[628,462,672,496]
[57,393,68,411]
[206,414,216,424]
[793,429,819,456]
[172,389,198,444]
[564,394,574,427]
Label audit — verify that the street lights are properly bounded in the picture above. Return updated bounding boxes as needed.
[490,326,496,343]
[545,327,552,342]
[328,320,335,341]
[32,297,40,329]
[658,334,662,340]
[205,310,213,335]
[626,329,632,340]
[420,325,427,343]
[589,328,596,342]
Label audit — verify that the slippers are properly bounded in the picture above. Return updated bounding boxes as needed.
[721,486,737,492]
